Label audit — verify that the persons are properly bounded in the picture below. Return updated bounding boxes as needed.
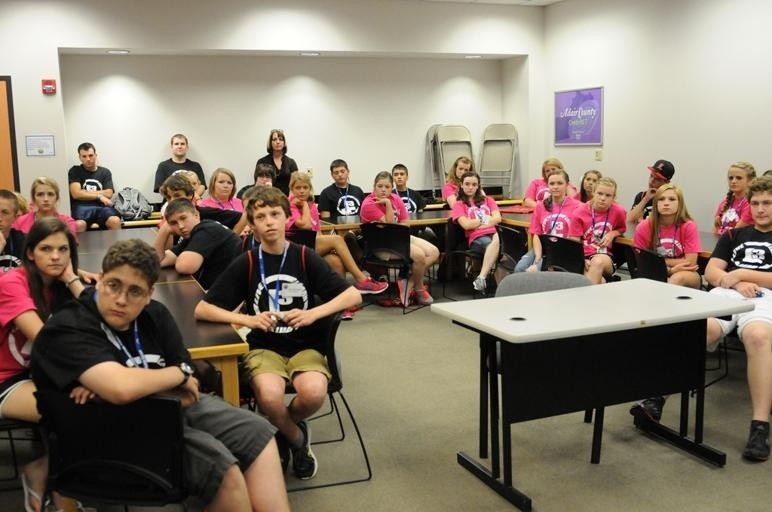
[288,172,388,320]
[576,171,601,202]
[1,189,30,267]
[256,129,298,195]
[452,173,502,292]
[154,135,207,202]
[631,175,771,462]
[68,144,122,232]
[160,175,248,240]
[443,157,487,210]
[360,172,439,306]
[200,168,248,215]
[318,160,363,218]
[515,169,582,272]
[13,177,78,249]
[237,164,276,196]
[634,185,702,289]
[195,187,362,480]
[1,217,87,512]
[154,199,250,279]
[626,160,685,224]
[524,158,578,210]
[391,163,426,212]
[29,239,290,511]
[712,162,756,238]
[572,178,628,286]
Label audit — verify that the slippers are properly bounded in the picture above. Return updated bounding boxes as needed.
[20,471,50,512]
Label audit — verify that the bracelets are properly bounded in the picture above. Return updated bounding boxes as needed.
[66,275,80,287]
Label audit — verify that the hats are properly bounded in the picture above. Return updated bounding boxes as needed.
[647,160,675,180]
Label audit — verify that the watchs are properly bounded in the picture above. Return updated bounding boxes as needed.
[175,362,193,385]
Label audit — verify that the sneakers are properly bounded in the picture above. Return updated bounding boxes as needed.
[291,421,318,480]
[629,395,666,425]
[354,276,389,295]
[414,288,433,305]
[473,277,486,295]
[397,280,413,307]
[274,431,290,472]
[742,419,771,461]
[341,310,353,319]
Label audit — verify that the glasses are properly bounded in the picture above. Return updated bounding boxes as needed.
[101,278,149,301]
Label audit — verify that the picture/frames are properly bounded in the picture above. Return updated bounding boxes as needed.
[553,86,604,147]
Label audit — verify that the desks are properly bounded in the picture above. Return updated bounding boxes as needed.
[76,228,249,410]
[429,276,755,512]
[318,204,534,250]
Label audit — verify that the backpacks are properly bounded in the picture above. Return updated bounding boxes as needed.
[112,187,152,220]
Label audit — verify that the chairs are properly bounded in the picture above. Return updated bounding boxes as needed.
[483,185,504,197]
[427,124,454,198]
[441,217,503,303]
[413,189,433,199]
[478,270,605,468]
[477,123,517,199]
[358,222,431,315]
[30,393,184,512]
[314,194,320,203]
[537,233,585,276]
[287,228,317,251]
[624,243,667,284]
[435,188,442,199]
[435,125,474,187]
[364,192,371,197]
[239,310,371,493]
